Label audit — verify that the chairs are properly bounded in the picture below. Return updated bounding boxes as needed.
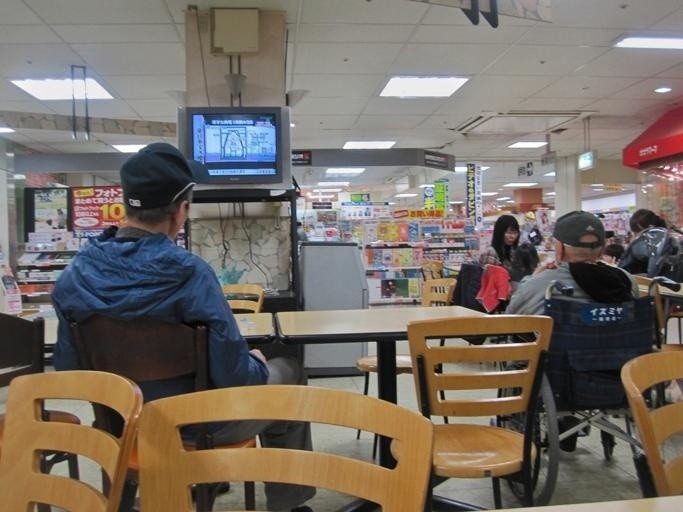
[0,249,683,512]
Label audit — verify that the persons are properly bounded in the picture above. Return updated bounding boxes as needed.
[656,215,667,229]
[478,214,520,270]
[504,210,640,453]
[618,207,683,312]
[541,211,550,230]
[601,230,633,265]
[509,240,541,282]
[52,208,65,230]
[50,142,317,511]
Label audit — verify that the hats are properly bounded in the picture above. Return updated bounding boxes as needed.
[552,210,605,248]
[118,143,210,210]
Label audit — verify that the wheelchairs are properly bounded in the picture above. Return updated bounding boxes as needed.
[490,275,681,506]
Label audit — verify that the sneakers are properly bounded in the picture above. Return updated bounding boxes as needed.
[190,482,230,502]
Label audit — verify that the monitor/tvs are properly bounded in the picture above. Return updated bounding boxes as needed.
[175,106,294,190]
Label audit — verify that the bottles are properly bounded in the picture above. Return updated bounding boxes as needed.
[420,218,480,266]
